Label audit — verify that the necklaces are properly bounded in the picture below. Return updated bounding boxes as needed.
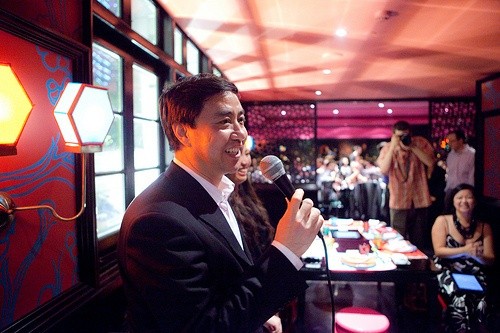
[452,213,476,239]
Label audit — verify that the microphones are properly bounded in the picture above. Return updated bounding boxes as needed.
[260,155,323,239]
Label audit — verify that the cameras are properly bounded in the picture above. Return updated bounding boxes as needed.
[398,134,412,145]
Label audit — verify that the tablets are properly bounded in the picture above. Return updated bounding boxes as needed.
[450,273,484,293]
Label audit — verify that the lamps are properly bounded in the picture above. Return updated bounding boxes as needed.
[53,81,113,154]
[0,62,33,156]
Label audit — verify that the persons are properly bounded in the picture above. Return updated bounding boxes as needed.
[118,72,324,333]
[431,183,495,333]
[376,121,436,247]
[437,129,476,215]
[225,145,275,272]
[251,141,390,227]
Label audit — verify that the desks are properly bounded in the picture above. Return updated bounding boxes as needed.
[295,218,428,333]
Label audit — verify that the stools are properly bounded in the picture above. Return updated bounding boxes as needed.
[334,306,390,333]
